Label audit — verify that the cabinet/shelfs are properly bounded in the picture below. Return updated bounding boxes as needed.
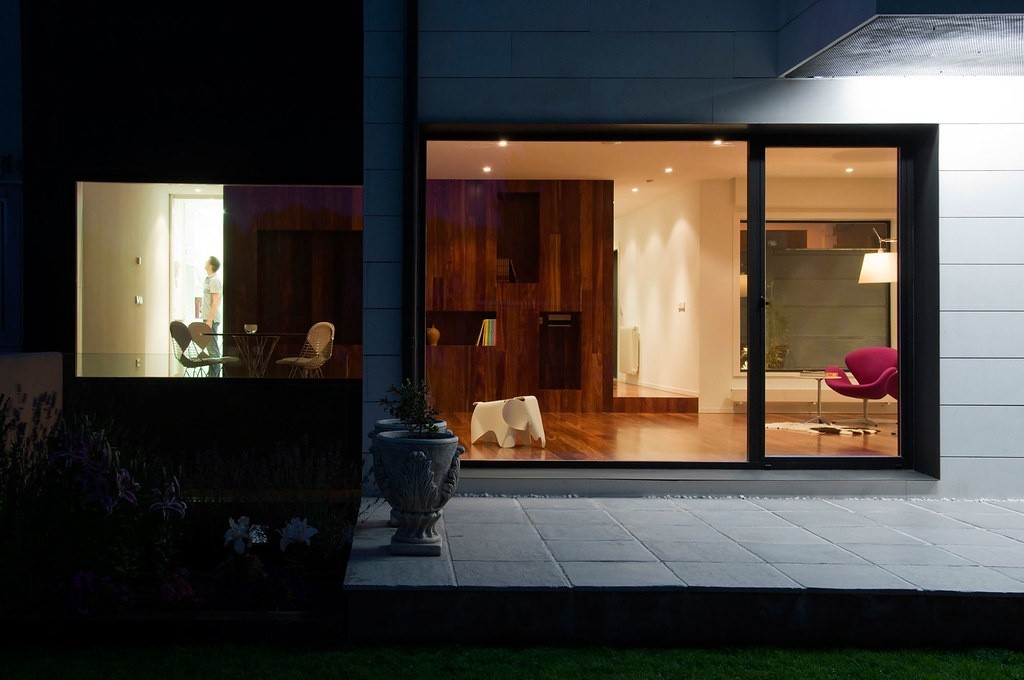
[223,180,614,411]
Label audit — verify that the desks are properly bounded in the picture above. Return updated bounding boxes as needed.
[200,333,307,378]
[799,376,842,428]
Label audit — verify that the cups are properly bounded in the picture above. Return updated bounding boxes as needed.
[244,324,258,334]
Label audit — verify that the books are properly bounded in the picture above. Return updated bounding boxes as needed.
[497,258,519,283]
[476,318,496,346]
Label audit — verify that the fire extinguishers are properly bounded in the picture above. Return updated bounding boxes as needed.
[196,298,200,318]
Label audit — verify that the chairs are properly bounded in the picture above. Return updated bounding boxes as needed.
[824,346,898,427]
[276,321,335,378]
[170,321,240,378]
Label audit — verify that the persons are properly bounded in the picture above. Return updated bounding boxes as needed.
[202,256,222,377]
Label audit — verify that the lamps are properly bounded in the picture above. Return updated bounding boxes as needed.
[859,227,897,284]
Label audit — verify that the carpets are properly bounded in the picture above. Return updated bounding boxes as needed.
[764,421,877,436]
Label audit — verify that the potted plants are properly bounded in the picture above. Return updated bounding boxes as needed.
[368,379,465,557]
